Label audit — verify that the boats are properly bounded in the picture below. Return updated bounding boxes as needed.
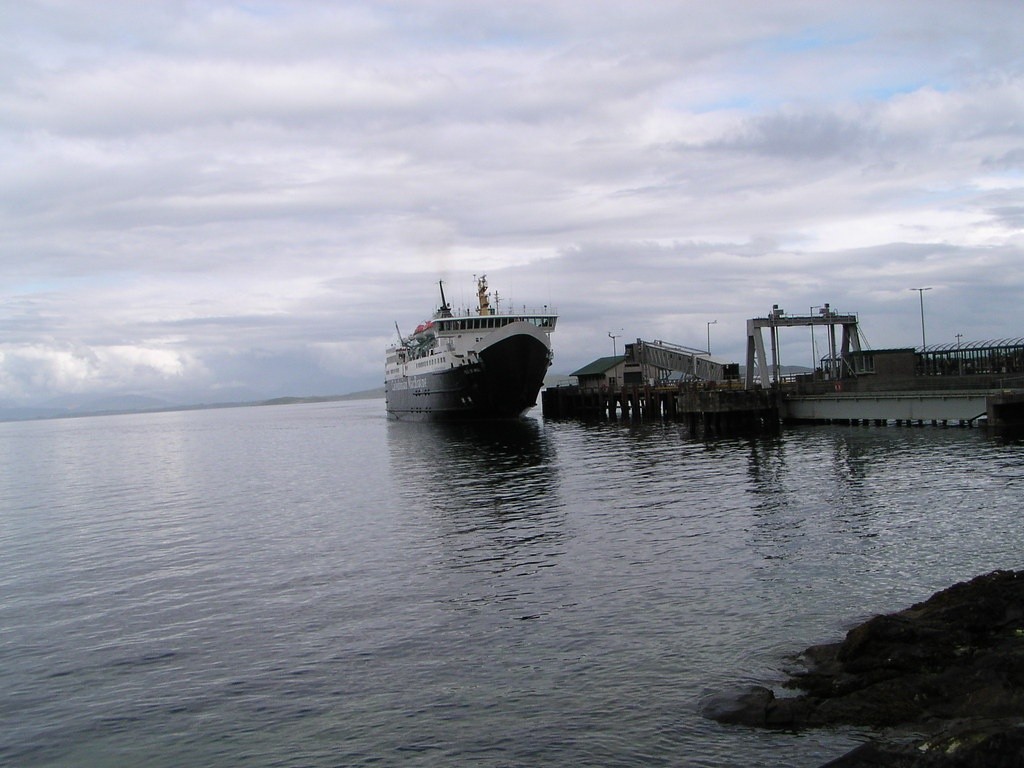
[381,273,562,422]
